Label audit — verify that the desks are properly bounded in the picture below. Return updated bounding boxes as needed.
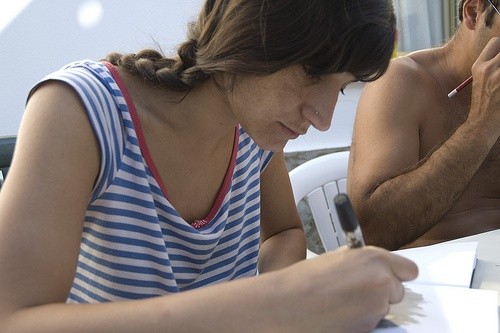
[371,229,500,333]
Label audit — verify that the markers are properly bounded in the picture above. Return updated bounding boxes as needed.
[334,193,365,249]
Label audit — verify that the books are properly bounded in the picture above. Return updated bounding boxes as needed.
[374,240,480,290]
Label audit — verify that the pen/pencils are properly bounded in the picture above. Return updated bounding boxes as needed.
[447,75,473,98]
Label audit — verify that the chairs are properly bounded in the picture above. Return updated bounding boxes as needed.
[289,151,351,260]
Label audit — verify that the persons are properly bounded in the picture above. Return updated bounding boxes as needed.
[1,0,419,333]
[346,0,500,253]
